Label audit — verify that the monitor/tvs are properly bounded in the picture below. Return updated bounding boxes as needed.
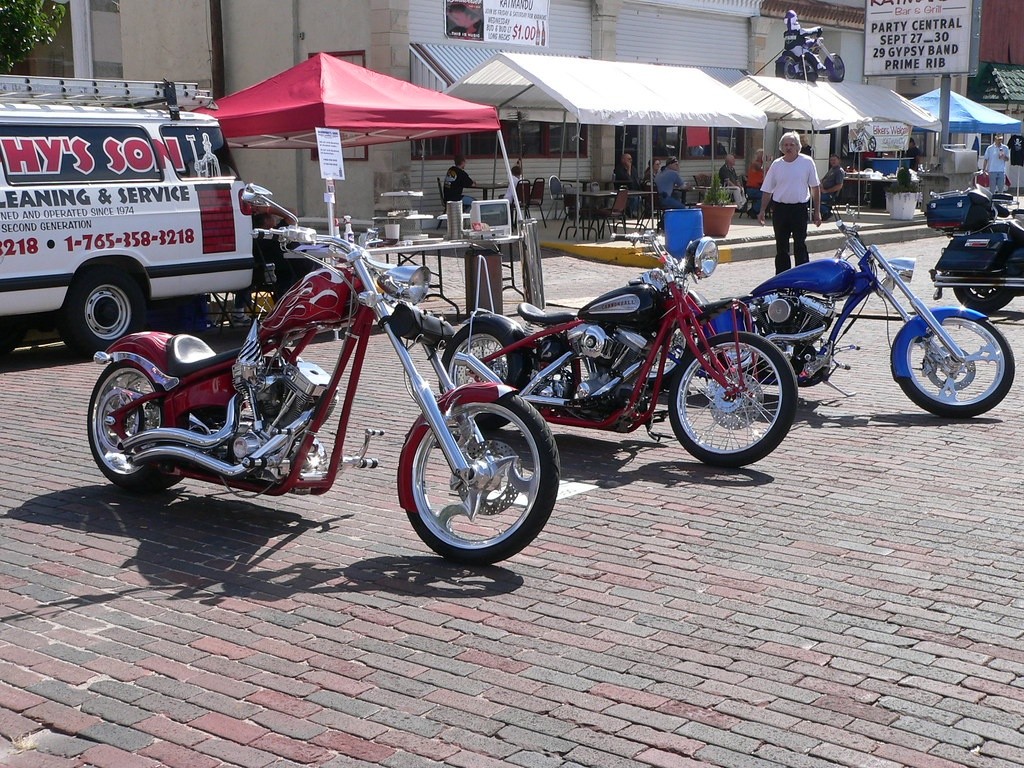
[470,198,511,239]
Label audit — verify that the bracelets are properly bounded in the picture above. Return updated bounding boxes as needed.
[267,207,271,213]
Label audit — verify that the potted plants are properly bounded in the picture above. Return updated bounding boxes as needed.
[882,158,921,221]
[696,164,738,238]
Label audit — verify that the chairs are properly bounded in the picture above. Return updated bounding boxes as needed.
[558,183,594,242]
[636,173,652,228]
[610,169,618,189]
[587,184,629,240]
[822,189,840,222]
[435,176,448,230]
[510,178,532,231]
[545,175,564,220]
[738,175,771,219]
[639,180,665,232]
[527,177,548,228]
[693,173,713,203]
[584,181,612,240]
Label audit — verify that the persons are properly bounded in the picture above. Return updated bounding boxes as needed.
[643,158,662,217]
[810,154,845,220]
[499,165,524,209]
[898,137,920,172]
[758,130,822,274]
[983,134,1009,194]
[613,154,642,218]
[654,155,690,231]
[230,204,321,325]
[443,156,477,229]
[799,134,815,159]
[719,154,752,211]
[783,9,826,72]
[746,150,772,218]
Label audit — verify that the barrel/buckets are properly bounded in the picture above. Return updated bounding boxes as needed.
[385,223,400,242]
[664,208,703,263]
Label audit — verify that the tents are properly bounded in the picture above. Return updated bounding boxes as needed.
[418,51,767,229]
[723,74,944,219]
[905,87,1022,206]
[187,52,524,273]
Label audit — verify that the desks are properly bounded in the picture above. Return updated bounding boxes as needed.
[566,190,658,239]
[463,182,510,200]
[675,186,738,207]
[366,235,526,323]
[560,178,634,219]
[843,178,920,182]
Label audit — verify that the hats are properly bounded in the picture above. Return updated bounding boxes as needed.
[995,135,1003,139]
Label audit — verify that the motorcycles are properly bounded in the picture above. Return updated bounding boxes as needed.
[85,181,564,568]
[921,184,1024,316]
[775,28,845,82]
[637,203,1016,419]
[437,230,799,468]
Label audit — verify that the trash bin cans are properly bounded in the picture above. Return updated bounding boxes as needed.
[464,244,504,319]
[663,208,706,267]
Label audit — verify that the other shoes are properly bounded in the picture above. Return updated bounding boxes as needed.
[657,221,664,229]
[748,208,758,219]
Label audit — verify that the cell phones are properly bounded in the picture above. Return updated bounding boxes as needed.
[1000,148,1003,152]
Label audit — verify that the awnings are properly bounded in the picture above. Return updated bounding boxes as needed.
[982,63,1024,105]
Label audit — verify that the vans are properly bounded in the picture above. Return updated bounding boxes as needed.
[0,72,260,358]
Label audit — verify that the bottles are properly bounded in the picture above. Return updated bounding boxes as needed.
[1000,148,1003,156]
